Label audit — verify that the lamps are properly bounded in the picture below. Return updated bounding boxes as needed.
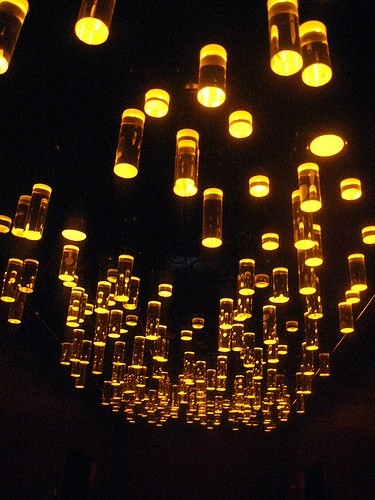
[0,0,375,433]
